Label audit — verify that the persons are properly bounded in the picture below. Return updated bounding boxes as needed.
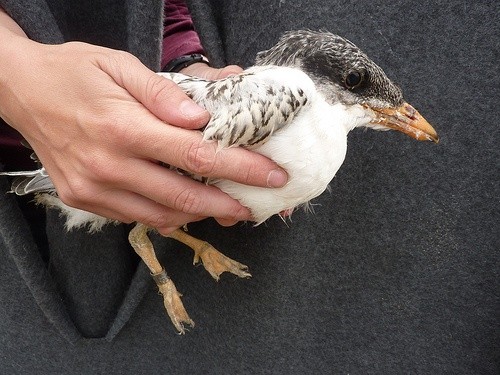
[0,0,295,235]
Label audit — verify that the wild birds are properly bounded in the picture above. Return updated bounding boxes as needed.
[0,27,439,336]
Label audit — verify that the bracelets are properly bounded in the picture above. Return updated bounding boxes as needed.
[162,52,210,72]
